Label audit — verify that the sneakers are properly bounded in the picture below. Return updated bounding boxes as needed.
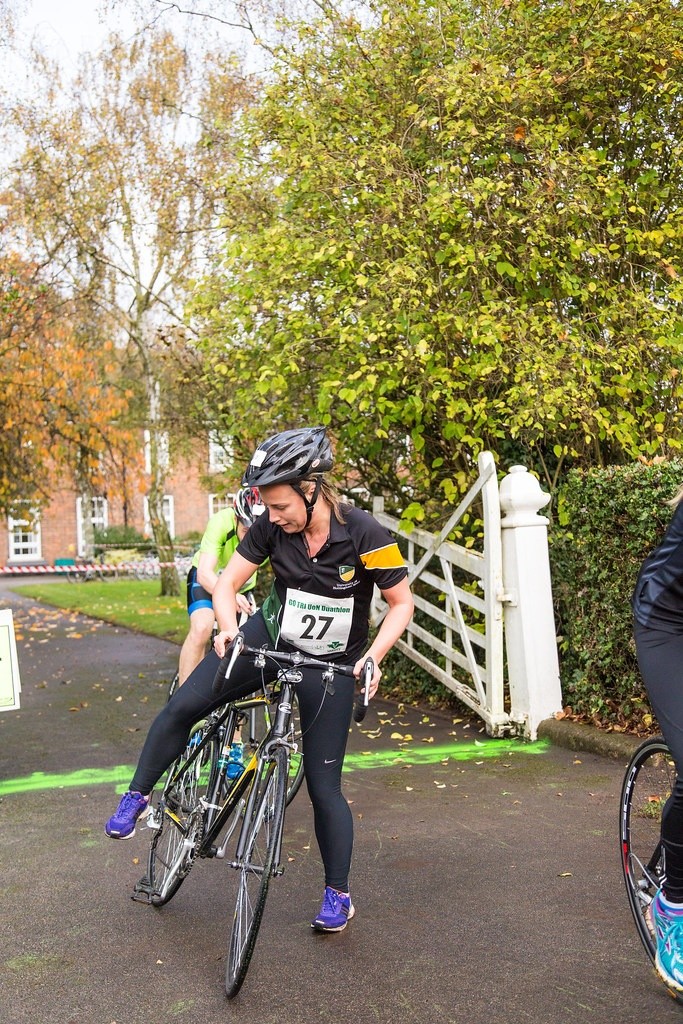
[190,724,212,768]
[105,789,150,841]
[311,888,356,932]
[224,739,246,780]
[643,887,682,992]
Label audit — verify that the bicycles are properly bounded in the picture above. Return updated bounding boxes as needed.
[617,732,682,1005]
[162,590,305,824]
[67,551,197,585]
[130,633,377,997]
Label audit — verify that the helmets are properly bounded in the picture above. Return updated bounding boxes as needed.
[234,487,268,529]
[240,424,335,492]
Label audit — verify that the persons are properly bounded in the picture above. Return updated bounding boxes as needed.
[176,481,268,781]
[628,489,683,1009]
[102,426,418,934]
